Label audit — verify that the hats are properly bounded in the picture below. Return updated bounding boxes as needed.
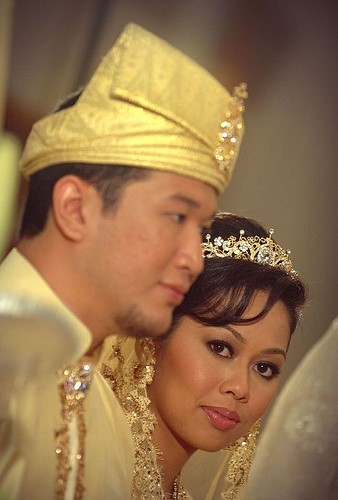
[16,22,249,195]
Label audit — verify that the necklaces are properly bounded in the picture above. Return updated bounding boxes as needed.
[173,476,178,500]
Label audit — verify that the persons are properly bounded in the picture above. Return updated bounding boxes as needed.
[0,21,308,500]
